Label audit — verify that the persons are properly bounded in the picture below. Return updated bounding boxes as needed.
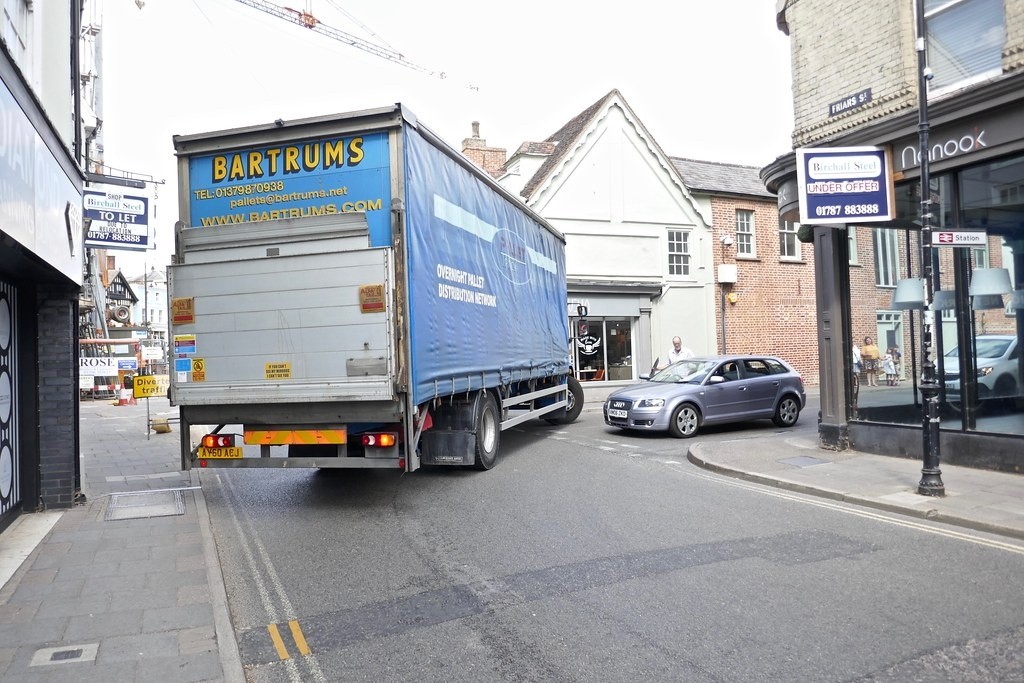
[850,342,861,388]
[654,336,695,377]
[878,344,902,386]
[861,336,881,386]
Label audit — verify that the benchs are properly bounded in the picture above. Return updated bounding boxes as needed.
[745,367,769,376]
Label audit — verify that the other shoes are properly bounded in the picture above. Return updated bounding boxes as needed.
[867,384,871,387]
[890,380,894,386]
[893,383,900,386]
[872,384,879,387]
[887,381,890,386]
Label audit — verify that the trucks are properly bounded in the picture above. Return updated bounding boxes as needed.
[166,103,584,475]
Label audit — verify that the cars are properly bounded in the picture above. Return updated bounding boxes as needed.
[603,355,806,438]
[920,333,1019,402]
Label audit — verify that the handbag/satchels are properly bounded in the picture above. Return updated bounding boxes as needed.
[856,361,863,368]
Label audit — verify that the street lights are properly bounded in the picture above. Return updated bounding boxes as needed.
[719,235,734,373]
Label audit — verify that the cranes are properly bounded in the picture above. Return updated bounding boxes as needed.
[239,1,480,93]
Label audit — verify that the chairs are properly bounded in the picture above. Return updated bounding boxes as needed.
[728,364,737,381]
[590,370,605,381]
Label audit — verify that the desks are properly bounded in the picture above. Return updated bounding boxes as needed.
[579,369,597,381]
